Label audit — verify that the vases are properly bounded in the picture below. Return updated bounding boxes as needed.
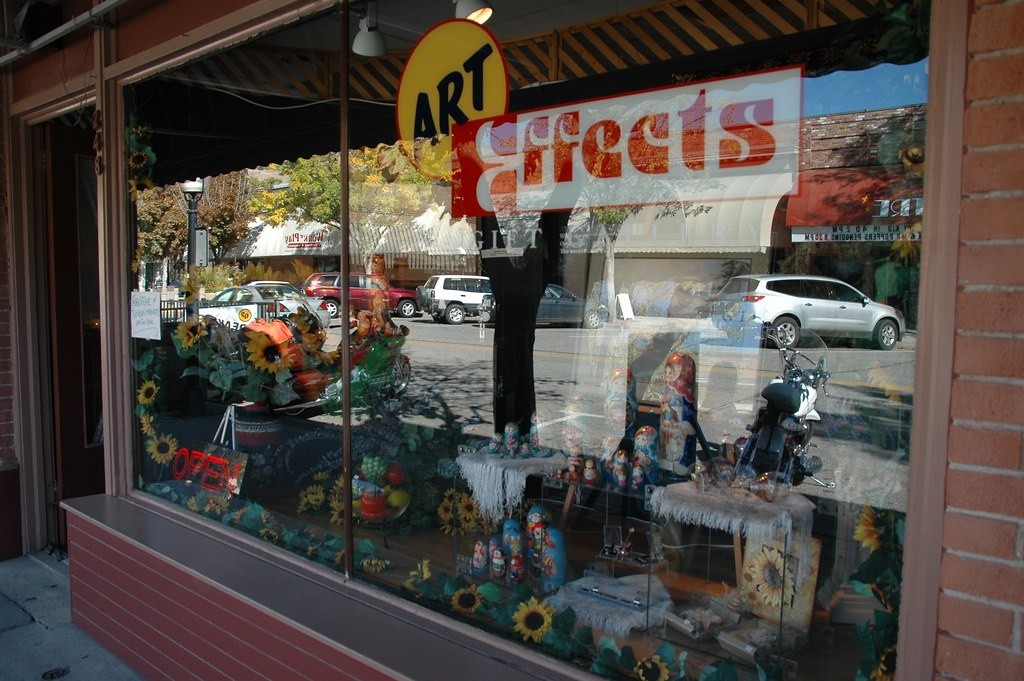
[229,415,283,455]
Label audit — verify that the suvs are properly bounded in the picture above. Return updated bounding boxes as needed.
[706,274,906,352]
[414,274,497,326]
[301,271,425,318]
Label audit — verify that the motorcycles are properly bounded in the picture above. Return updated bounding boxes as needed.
[731,315,838,492]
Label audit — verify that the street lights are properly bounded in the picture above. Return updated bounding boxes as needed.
[179,178,207,325]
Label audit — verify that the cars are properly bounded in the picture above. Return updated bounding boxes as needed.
[479,285,610,329]
[201,279,331,337]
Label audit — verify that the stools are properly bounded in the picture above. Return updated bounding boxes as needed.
[455,446,567,545]
[652,480,814,671]
